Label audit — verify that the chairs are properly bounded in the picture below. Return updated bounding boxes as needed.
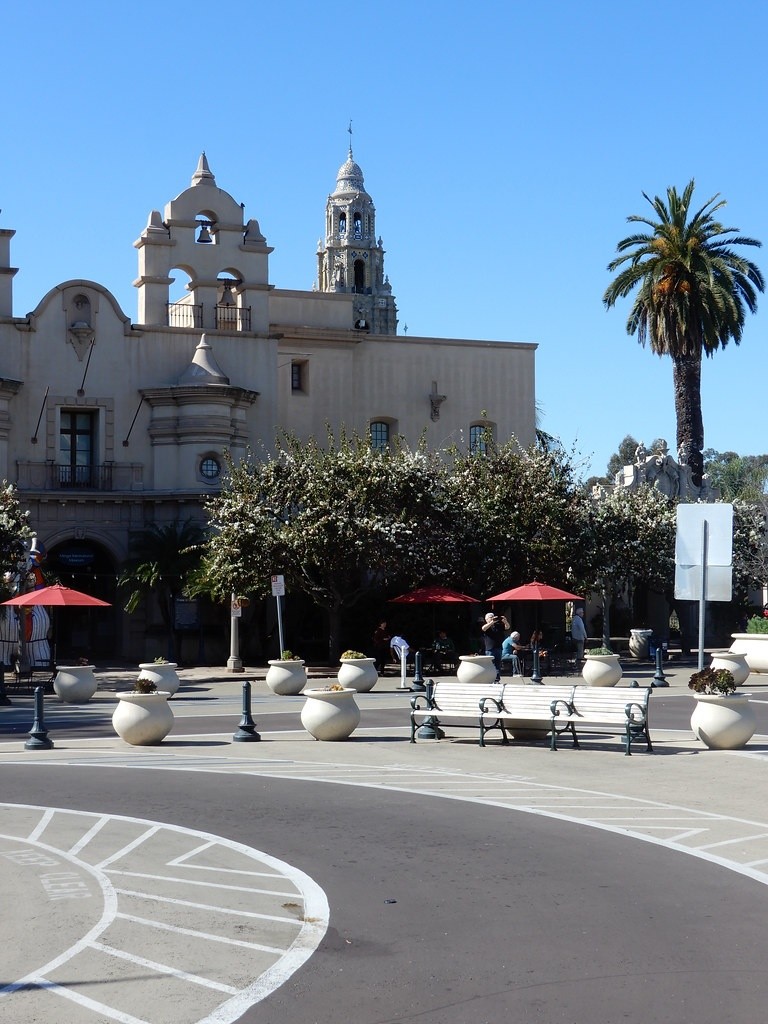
[10,654,33,691]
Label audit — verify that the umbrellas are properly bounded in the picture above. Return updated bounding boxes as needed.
[486,577,586,676]
[386,582,482,671]
[0,579,112,681]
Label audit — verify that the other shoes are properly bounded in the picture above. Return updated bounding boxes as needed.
[513,673,523,677]
[494,680,500,684]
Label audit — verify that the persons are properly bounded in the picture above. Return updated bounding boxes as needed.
[738,611,748,633]
[429,631,454,672]
[372,619,386,677]
[501,629,520,654]
[482,613,510,684]
[571,607,587,659]
[501,631,529,674]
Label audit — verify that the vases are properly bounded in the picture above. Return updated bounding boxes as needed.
[629,629,653,657]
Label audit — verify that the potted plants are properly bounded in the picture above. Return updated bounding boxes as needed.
[687,667,757,750]
[582,648,623,687]
[112,679,175,745]
[456,653,497,684]
[338,650,378,693]
[710,652,750,686]
[265,650,307,695]
[15,656,30,674]
[53,658,97,704]
[300,684,360,740]
[137,656,180,699]
[729,614,768,672]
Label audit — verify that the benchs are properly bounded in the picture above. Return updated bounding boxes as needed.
[408,679,654,755]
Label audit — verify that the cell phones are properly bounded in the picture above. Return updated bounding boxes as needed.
[496,616,503,620]
